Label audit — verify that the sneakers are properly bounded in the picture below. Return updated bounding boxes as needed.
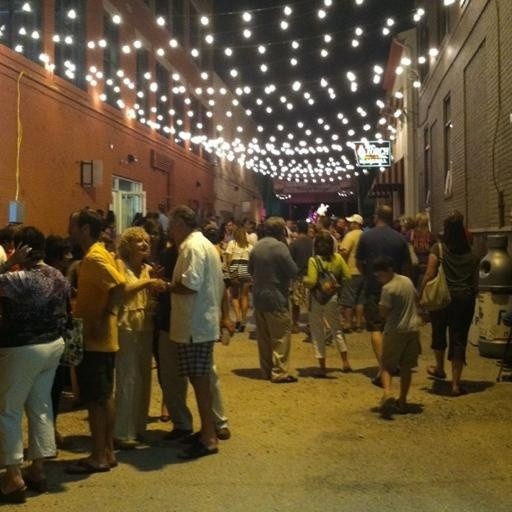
[54,431,63,444]
[292,324,298,333]
[312,369,326,376]
[427,366,445,377]
[381,392,394,409]
[163,430,192,440]
[239,322,245,332]
[392,399,409,414]
[235,319,241,330]
[217,429,230,439]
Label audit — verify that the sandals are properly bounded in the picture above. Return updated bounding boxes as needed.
[342,326,352,333]
[352,325,362,333]
[161,404,170,421]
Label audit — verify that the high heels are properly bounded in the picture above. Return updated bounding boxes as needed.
[26,478,48,493]
[0,485,27,502]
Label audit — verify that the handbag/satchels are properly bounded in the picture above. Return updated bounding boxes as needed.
[60,317,84,368]
[419,263,452,312]
[310,270,341,305]
[229,268,238,280]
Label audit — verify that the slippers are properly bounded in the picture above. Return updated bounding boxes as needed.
[178,441,218,458]
[183,433,200,441]
[64,456,117,474]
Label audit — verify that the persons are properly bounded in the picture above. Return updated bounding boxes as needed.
[418,208,481,395]
[226,203,431,379]
[0,204,229,495]
[371,256,422,414]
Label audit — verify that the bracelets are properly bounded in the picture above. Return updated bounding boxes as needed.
[165,278,173,291]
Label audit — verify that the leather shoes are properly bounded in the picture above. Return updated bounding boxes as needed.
[280,375,297,383]
[113,439,151,450]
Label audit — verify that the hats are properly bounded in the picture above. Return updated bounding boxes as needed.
[345,213,364,225]
